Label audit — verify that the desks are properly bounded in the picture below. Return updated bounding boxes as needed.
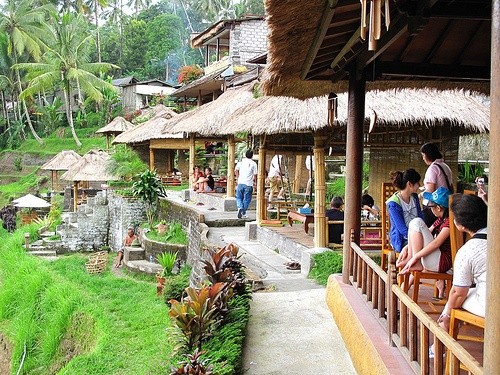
[287,211,314,233]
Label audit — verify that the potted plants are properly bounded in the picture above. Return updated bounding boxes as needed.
[155,250,179,285]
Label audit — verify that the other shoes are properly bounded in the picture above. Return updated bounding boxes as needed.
[428,343,445,358]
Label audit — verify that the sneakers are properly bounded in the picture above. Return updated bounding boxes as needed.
[237,207,248,218]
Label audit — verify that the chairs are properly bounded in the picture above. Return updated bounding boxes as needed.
[381,182,485,375]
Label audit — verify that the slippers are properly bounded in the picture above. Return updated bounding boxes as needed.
[286,261,301,270]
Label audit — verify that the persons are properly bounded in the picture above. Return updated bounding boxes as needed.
[361,194,382,227]
[235,150,258,218]
[396,186,466,276]
[429,193,488,358]
[305,155,327,194]
[325,196,344,249]
[190,165,214,194]
[385,168,445,302]
[268,155,288,210]
[418,142,453,237]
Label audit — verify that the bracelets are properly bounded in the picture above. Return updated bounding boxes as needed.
[445,314,450,317]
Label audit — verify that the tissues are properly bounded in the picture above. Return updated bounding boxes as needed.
[300,203,312,215]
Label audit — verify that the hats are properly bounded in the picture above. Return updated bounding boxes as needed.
[423,186,452,207]
[330,196,345,207]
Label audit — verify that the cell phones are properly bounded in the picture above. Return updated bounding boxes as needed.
[419,187,425,189]
